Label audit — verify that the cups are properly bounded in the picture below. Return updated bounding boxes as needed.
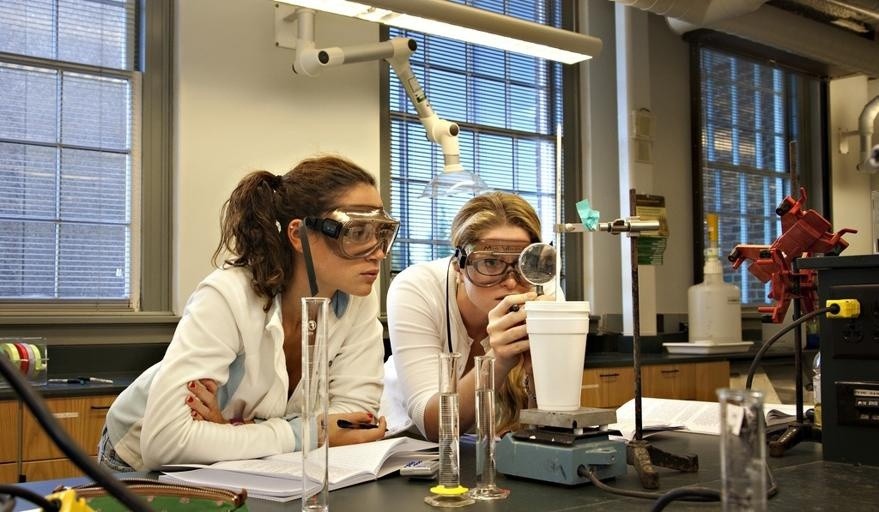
[525,300,591,411]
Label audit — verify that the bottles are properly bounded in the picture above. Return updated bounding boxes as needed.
[812,345,822,427]
[716,388,767,512]
[300,297,330,512]
[426,352,511,508]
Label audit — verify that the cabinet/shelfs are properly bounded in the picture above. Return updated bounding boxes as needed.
[639,364,729,407]
[1,398,118,491]
[580,366,639,409]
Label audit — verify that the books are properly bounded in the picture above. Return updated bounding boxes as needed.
[158,436,439,503]
[608,395,815,441]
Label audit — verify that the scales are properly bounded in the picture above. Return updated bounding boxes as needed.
[496,408,626,485]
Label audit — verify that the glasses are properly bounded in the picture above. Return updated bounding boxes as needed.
[299,206,400,258]
[456,238,535,289]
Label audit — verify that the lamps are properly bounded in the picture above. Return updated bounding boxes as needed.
[270,1,604,66]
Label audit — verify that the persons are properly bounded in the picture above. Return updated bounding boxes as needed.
[97,155,386,471]
[380,191,566,441]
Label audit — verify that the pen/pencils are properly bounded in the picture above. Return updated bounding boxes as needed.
[48,376,114,384]
[335,422,391,430]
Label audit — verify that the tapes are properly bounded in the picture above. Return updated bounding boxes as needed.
[1,343,41,379]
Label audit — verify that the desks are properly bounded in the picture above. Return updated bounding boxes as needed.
[0,430,878,512]
